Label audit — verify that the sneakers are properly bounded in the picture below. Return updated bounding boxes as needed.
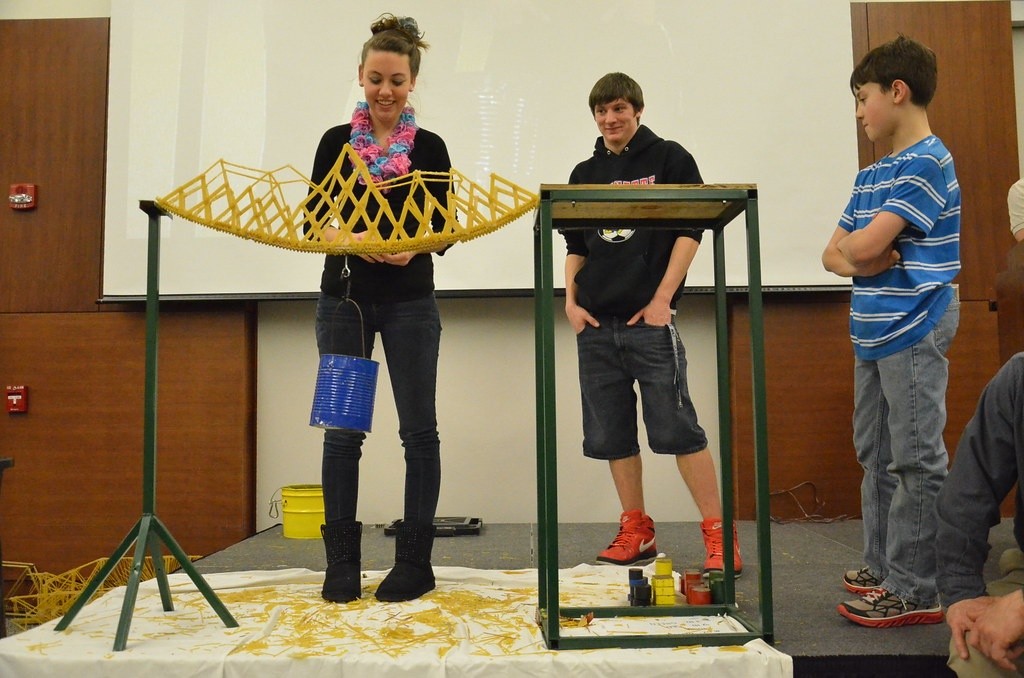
[595,509,657,566]
[843,566,887,597]
[700,518,742,580]
[838,588,943,629]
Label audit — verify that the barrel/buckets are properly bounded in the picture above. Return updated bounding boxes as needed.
[309,299,381,433]
[281,483,325,539]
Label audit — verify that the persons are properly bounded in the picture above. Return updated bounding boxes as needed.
[820,32,962,628]
[1005,172,1024,243]
[934,351,1024,677]
[558,70,745,579]
[300,12,460,603]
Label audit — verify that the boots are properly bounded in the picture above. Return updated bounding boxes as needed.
[375,522,435,603]
[320,521,363,604]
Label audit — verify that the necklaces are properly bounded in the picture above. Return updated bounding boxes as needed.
[348,100,418,194]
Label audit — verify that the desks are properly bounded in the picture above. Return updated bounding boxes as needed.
[533,184,776,652]
